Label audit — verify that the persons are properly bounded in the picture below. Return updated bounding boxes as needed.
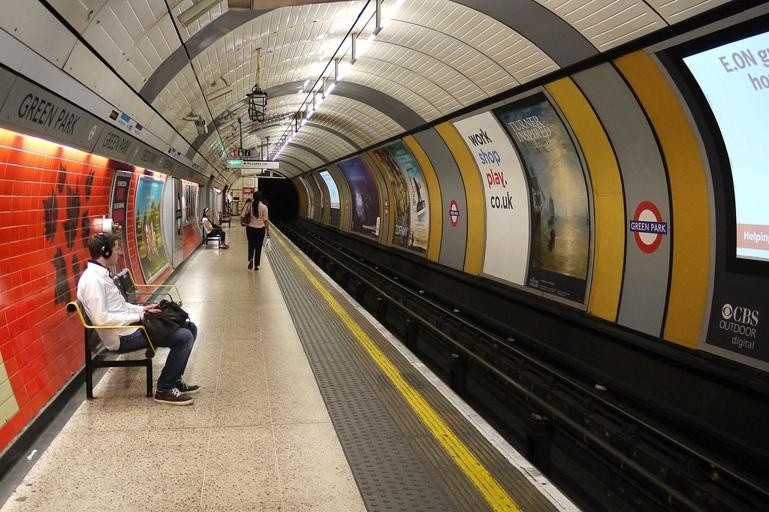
[201,207,229,248]
[76,231,201,405]
[241,190,270,270]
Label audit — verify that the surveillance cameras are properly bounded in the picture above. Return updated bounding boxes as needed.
[195,114,209,135]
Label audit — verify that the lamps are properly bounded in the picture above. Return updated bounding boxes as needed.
[245,49,268,122]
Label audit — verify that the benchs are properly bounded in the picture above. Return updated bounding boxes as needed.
[64,266,183,398]
[200,211,232,250]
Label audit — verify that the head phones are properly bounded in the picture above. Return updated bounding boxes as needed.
[97,232,112,259]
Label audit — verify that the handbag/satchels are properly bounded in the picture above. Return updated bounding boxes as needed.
[240,203,251,227]
[140,297,190,348]
[263,236,273,255]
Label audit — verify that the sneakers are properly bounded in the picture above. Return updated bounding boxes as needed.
[247,259,260,270]
[176,378,200,395]
[219,243,230,249]
[153,387,195,406]
